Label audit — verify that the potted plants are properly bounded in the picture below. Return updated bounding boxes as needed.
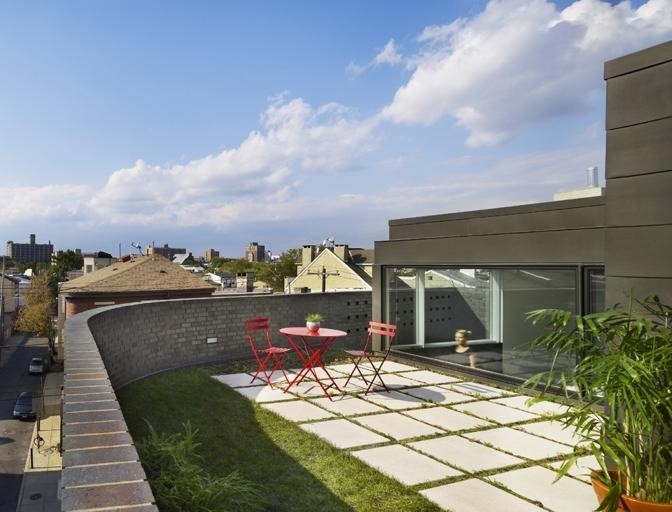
[515,285,672,512]
[304,312,324,332]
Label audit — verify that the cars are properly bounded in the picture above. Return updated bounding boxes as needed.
[29,358,45,375]
[13,391,36,419]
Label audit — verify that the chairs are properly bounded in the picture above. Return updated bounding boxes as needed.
[244,318,291,390]
[344,321,398,396]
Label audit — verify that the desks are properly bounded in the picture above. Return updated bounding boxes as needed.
[278,327,348,401]
